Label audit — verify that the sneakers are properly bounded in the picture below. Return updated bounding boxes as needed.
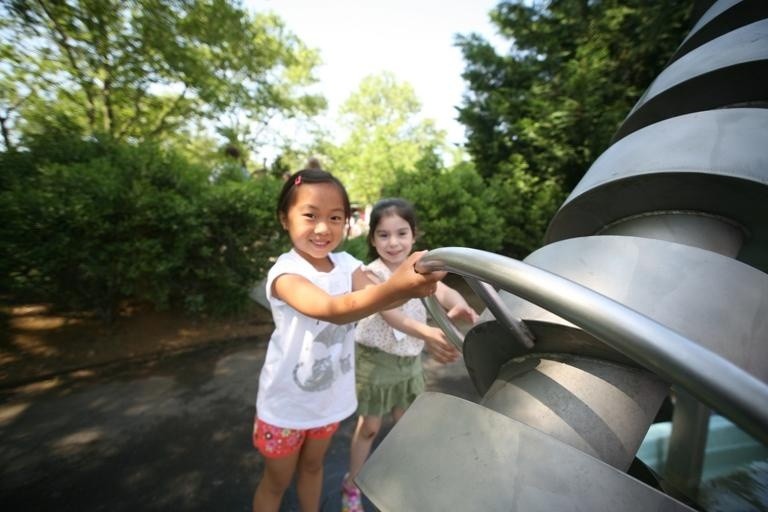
[341,472,363,512]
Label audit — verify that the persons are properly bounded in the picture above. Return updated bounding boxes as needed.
[207,145,252,183]
[304,156,323,170]
[345,203,374,241]
[340,197,481,512]
[251,169,448,511]
